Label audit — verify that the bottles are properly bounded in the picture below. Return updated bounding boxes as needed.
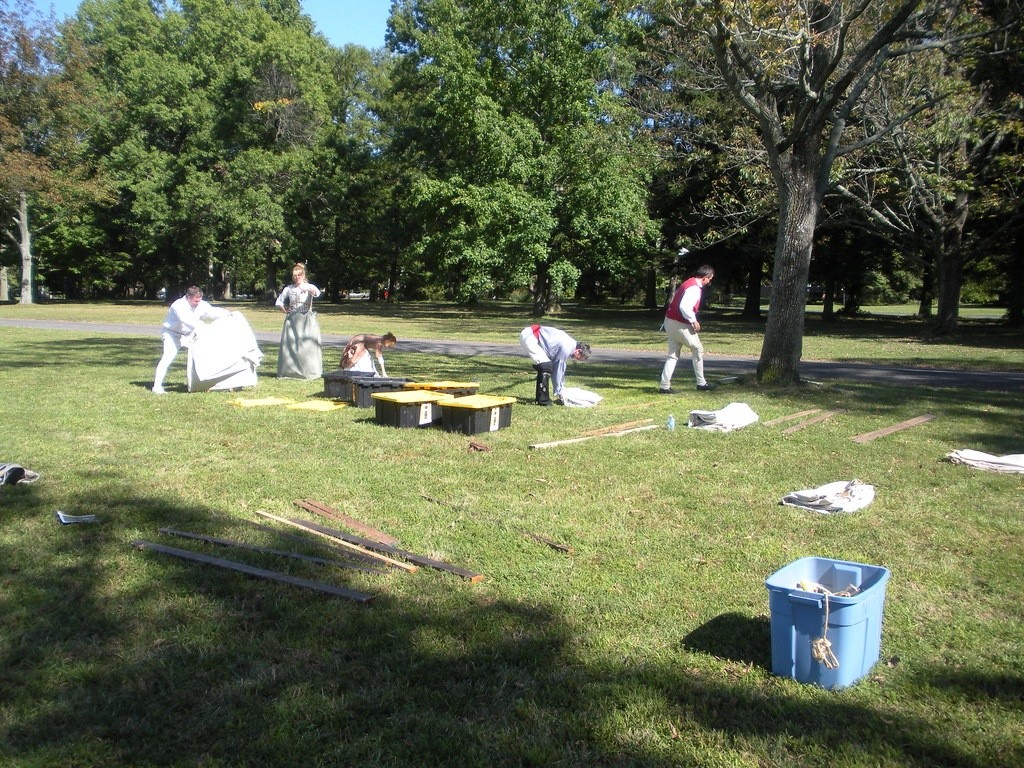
[667,414,675,430]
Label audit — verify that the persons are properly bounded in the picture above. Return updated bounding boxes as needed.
[153,286,229,394]
[275,263,321,380]
[339,332,396,378]
[519,325,592,406]
[659,265,718,393]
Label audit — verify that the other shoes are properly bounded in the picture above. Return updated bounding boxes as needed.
[697,383,717,391]
[550,402,557,406]
[152,389,166,394]
[659,387,679,394]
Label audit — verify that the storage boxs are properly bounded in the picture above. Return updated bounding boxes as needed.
[437,394,518,436]
[371,390,454,428]
[349,376,419,409]
[403,381,479,398]
[321,370,376,398]
[765,556,892,692]
